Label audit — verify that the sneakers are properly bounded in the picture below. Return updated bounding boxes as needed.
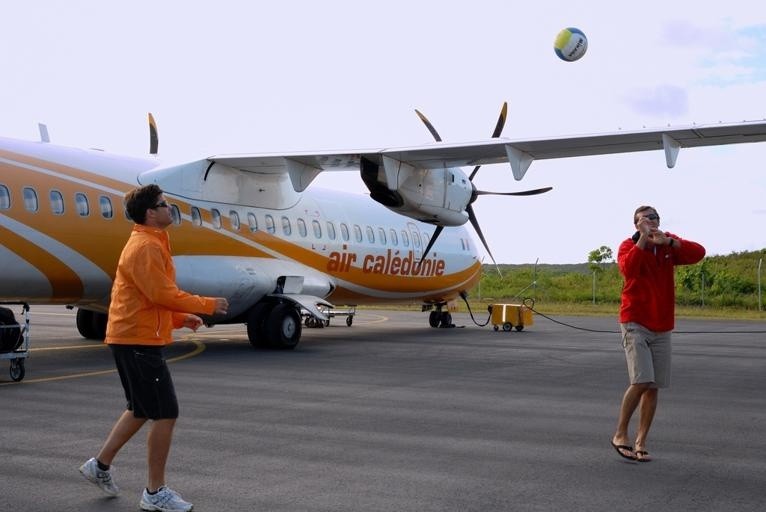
[79,457,119,495]
[139,484,193,512]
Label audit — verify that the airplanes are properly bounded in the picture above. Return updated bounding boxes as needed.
[0,100,766,350]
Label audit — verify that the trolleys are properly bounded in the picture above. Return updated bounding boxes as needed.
[301,303,355,327]
[0,301,30,382]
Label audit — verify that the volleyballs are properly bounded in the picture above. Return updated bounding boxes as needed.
[554,27,588,60]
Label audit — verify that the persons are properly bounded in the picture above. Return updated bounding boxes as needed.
[79,185,230,512]
[612,206,705,462]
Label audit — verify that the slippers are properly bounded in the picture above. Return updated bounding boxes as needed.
[611,439,653,462]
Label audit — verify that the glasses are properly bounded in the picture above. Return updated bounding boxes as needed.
[153,200,168,208]
[642,214,659,220]
